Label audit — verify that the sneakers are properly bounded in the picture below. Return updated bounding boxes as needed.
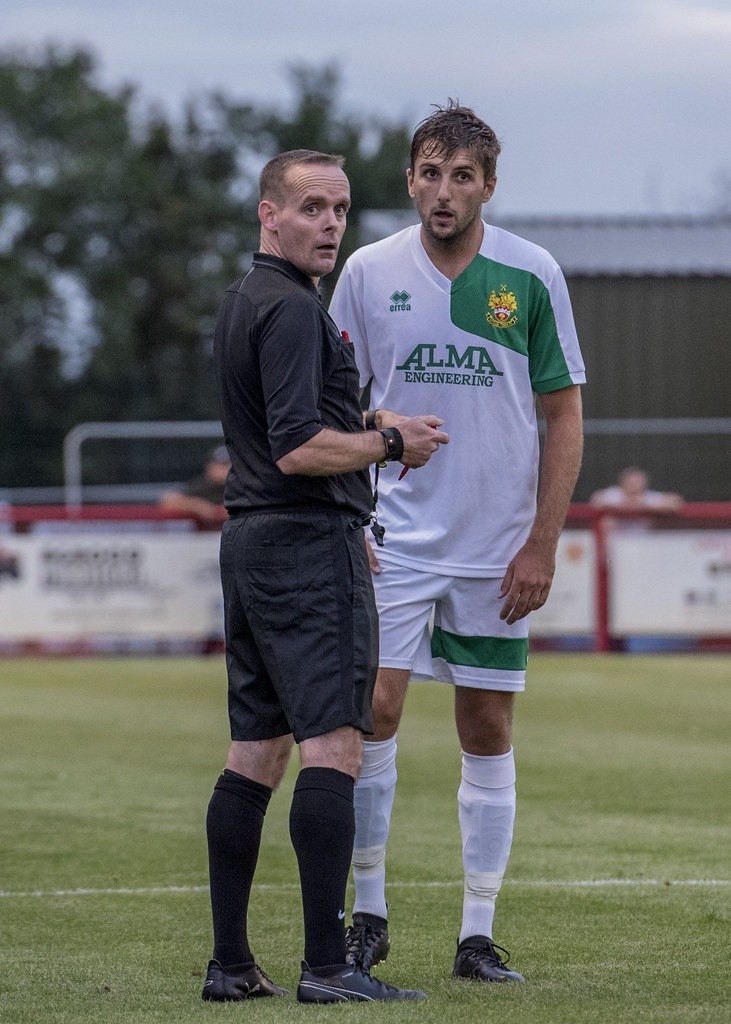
[202,959,290,1002]
[297,960,428,1006]
[343,901,390,974]
[451,935,525,983]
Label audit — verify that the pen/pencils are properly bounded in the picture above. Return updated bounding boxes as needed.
[341,330,350,344]
[397,426,437,481]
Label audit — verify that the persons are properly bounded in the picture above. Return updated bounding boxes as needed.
[161,450,232,654]
[196,147,425,1004]
[327,97,584,982]
[592,469,685,517]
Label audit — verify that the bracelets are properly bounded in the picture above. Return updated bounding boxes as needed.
[365,408,382,429]
[375,424,405,467]
